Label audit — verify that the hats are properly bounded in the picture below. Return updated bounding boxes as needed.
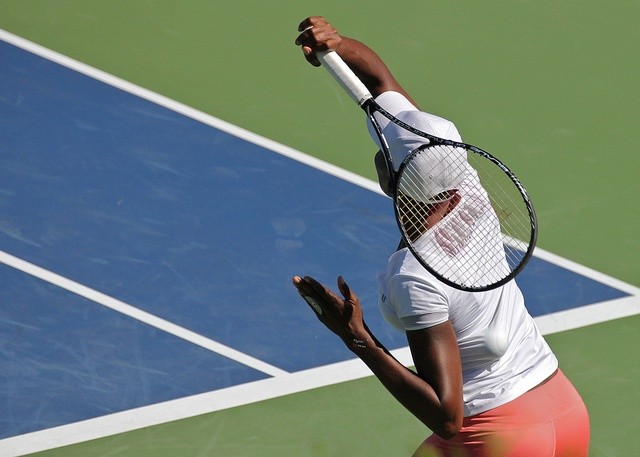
[366,89,468,204]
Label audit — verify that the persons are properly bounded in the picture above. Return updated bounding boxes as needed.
[293,17,590,456]
